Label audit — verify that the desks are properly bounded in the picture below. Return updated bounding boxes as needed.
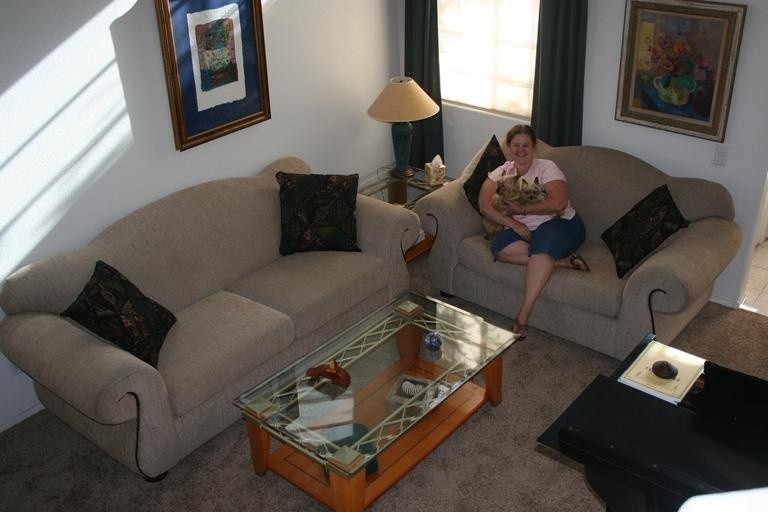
[357,162,456,262]
[535,333,768,511]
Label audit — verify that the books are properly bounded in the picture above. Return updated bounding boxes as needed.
[617,339,704,406]
[296,378,354,429]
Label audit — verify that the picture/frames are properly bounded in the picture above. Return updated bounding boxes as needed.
[386,373,440,411]
[613,0,749,144]
[153,0,273,153]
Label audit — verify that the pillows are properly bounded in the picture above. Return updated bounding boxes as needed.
[462,133,508,219]
[274,170,363,256]
[61,258,178,370]
[598,183,691,279]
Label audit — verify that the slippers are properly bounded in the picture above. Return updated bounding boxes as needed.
[569,251,590,272]
[511,318,528,341]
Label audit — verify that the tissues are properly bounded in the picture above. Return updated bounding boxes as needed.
[424,154,446,187]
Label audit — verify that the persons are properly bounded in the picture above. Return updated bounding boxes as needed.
[479,125,588,343]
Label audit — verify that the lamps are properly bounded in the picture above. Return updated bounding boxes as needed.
[365,76,441,178]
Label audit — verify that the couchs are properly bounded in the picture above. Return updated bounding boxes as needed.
[413,129,744,362]
[0,154,421,483]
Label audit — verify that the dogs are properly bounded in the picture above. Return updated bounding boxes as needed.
[513,176,547,215]
[484,169,520,241]
